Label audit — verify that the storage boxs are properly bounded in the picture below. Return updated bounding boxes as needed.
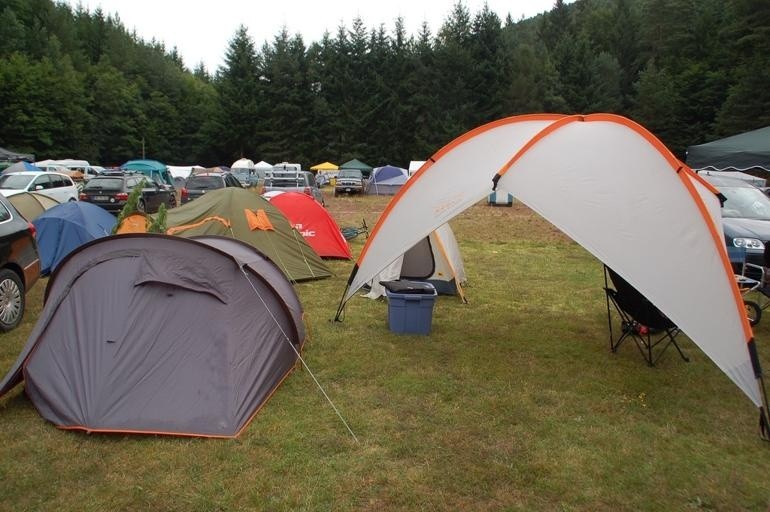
[385,279,438,336]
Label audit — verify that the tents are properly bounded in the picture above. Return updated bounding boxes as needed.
[1,232,309,439]
[148,185,337,283]
[365,164,409,195]
[120,159,177,192]
[268,190,352,260]
[360,220,469,302]
[31,200,120,277]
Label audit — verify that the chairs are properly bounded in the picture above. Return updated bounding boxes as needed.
[602,263,690,368]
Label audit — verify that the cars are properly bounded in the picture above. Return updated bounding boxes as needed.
[0,170,79,332]
[31,159,259,214]
[262,171,325,207]
[697,173,770,276]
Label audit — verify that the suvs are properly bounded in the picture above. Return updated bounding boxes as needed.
[334,170,364,197]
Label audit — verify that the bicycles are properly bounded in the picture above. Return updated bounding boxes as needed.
[340,219,369,240]
